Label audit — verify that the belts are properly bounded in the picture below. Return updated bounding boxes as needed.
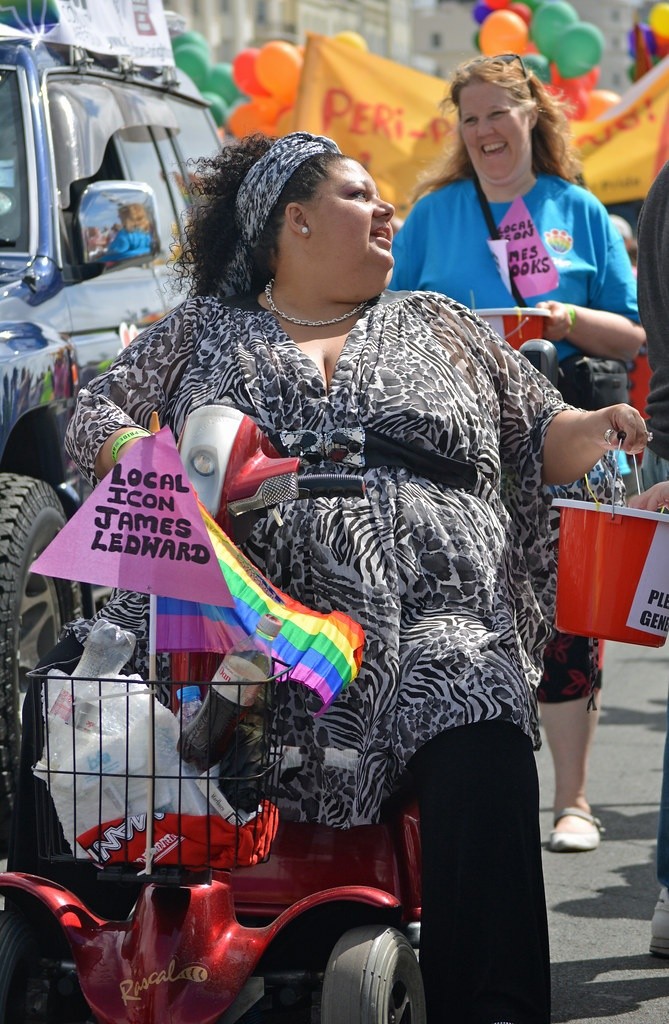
[267,428,477,495]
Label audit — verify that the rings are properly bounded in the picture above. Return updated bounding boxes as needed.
[604,429,614,445]
[646,431,653,441]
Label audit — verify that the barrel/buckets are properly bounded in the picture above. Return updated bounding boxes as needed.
[552,431,669,648]
[473,307,550,351]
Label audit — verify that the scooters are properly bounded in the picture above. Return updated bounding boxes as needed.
[0,406,428,1024]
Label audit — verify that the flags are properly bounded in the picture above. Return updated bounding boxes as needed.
[157,484,364,716]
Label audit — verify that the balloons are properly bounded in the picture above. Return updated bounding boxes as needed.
[172,29,368,136]
[471,0,622,118]
[627,0,669,82]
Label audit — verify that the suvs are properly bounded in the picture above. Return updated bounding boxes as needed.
[0,35,239,859]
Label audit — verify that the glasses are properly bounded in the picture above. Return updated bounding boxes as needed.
[456,52,526,82]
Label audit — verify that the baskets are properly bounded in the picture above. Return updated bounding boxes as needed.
[29,657,298,880]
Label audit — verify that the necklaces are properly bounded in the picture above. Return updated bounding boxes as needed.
[265,279,368,326]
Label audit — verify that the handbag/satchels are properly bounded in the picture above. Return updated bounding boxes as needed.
[559,353,629,412]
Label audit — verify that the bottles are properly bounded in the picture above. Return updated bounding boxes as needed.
[176,615,283,768]
[33,620,135,781]
[177,686,204,727]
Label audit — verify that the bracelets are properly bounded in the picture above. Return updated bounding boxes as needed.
[564,303,575,333]
[112,430,148,463]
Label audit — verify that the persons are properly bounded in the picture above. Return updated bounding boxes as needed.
[64,134,647,1024]
[389,59,645,471]
[2,347,68,430]
[631,158,669,957]
[94,204,152,261]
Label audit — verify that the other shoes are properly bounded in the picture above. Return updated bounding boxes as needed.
[550,808,605,852]
[649,887,669,954]
